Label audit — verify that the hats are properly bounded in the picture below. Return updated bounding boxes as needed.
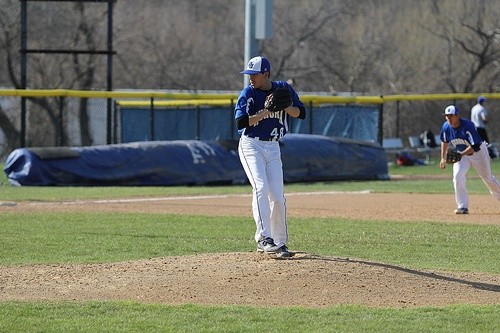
[240,56,270,74]
[477,97,484,103]
[442,105,459,115]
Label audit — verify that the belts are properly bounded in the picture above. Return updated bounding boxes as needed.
[470,148,480,154]
[249,135,272,141]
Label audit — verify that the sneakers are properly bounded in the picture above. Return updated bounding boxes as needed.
[276,245,295,257]
[257,238,282,253]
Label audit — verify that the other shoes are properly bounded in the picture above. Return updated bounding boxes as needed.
[454,208,469,214]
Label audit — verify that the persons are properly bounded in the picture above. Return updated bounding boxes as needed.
[438,102,500,215]
[469,97,498,160]
[230,55,307,254]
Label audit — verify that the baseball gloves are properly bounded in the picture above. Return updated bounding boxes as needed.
[264,88,292,112]
[446,149,461,163]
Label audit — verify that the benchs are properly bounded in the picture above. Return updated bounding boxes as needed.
[383,131,440,165]
